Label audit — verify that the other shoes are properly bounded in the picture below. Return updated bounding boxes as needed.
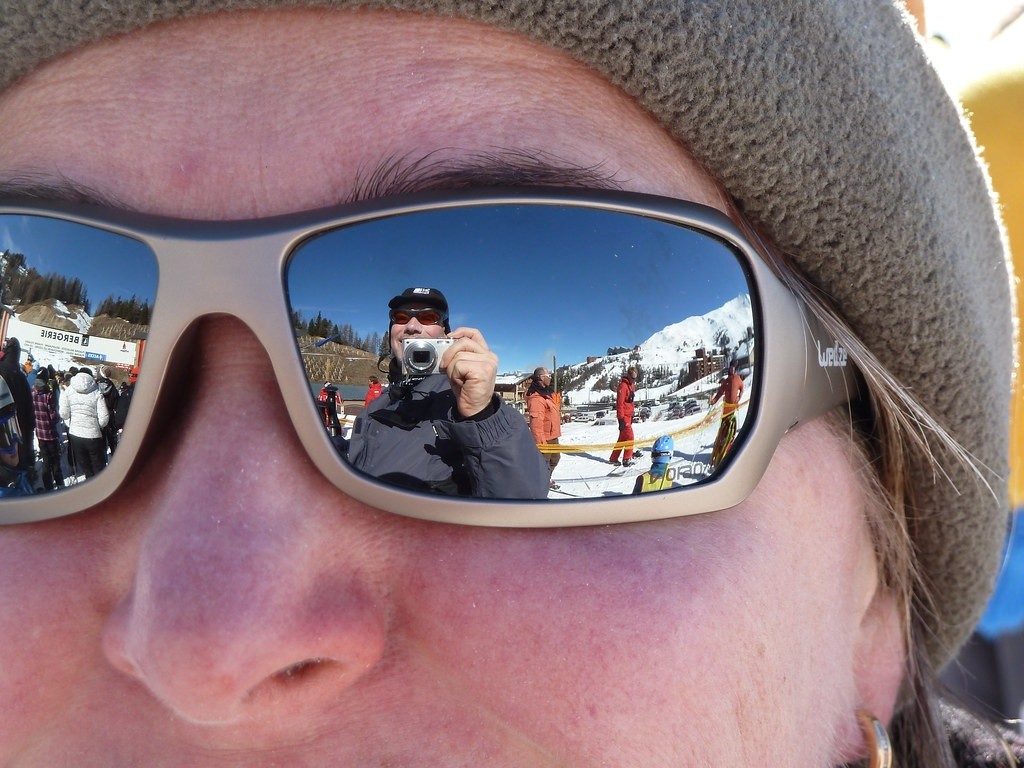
[609,460,621,465]
[549,480,560,491]
[623,458,639,466]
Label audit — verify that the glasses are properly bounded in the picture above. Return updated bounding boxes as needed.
[389,308,446,325]
[536,374,552,379]
[0,208,857,527]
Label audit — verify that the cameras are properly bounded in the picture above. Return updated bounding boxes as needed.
[401,337,457,375]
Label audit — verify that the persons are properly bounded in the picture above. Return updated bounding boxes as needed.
[0,0,1016,768]
[318,288,746,499]
[0,337,141,497]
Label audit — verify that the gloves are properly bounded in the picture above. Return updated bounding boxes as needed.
[618,419,625,430]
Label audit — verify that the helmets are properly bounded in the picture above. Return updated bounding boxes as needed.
[650,435,674,464]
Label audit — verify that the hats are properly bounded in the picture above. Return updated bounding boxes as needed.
[388,287,449,329]
[730,359,739,368]
[100,365,111,379]
[129,367,139,382]
[0,0,1013,706]
